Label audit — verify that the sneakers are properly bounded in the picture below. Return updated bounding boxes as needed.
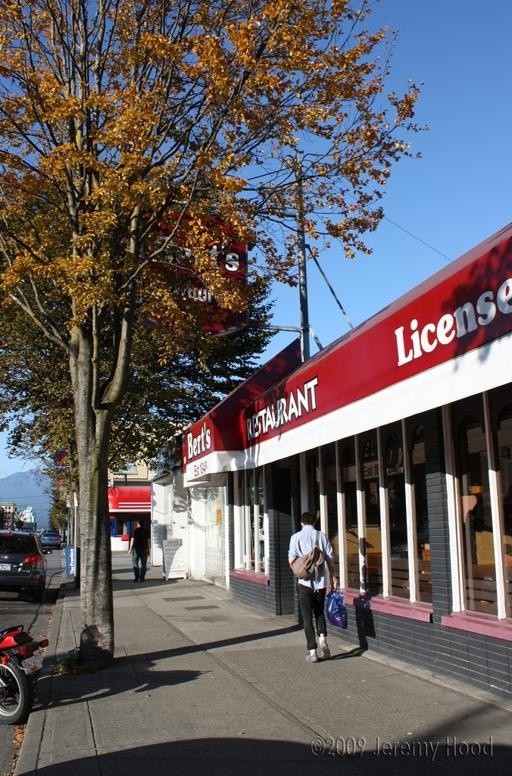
[321,641,332,661]
[133,576,145,583]
[305,652,318,663]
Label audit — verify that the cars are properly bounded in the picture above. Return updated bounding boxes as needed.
[0,529,62,598]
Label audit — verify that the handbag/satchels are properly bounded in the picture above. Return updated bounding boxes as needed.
[326,589,349,629]
[290,547,325,582]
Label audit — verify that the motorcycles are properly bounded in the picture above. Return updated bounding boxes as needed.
[0,624,49,725]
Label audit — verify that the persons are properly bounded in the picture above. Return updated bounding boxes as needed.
[287,510,339,664]
[126,520,151,583]
